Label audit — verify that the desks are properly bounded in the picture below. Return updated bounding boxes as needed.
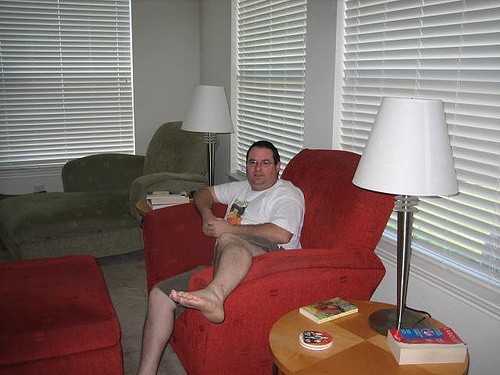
[136,196,194,230]
[268,299,470,375]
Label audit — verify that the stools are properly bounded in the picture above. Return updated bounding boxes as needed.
[0,255,124,375]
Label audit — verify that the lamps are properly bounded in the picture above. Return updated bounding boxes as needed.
[180,85,234,186]
[352,96,458,336]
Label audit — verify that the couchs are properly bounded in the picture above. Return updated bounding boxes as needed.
[0,121,218,266]
[142,149,395,375]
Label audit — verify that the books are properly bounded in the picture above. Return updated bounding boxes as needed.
[146,191,190,211]
[387,327,468,366]
[297,296,360,325]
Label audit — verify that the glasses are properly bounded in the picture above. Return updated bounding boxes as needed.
[247,160,274,167]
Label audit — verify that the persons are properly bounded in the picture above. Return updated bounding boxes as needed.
[135,140,306,375]
